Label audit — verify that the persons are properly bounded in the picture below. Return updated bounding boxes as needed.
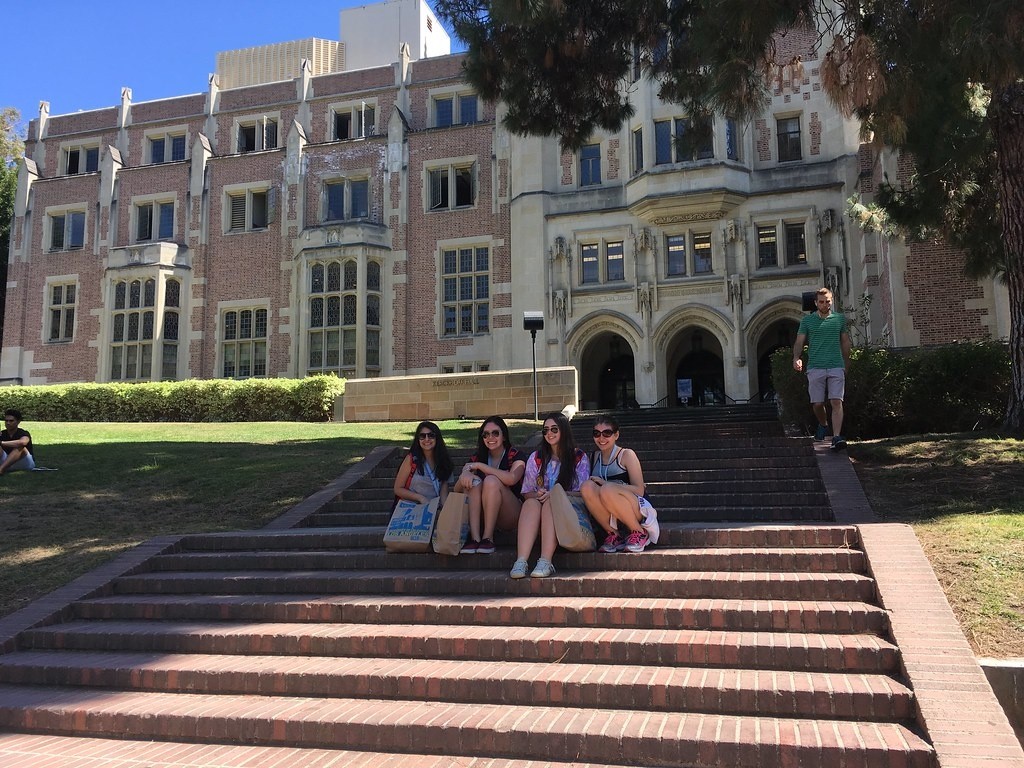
[0,409,35,473]
[580,414,651,554]
[509,413,590,579]
[454,415,528,555]
[393,421,454,531]
[793,287,850,450]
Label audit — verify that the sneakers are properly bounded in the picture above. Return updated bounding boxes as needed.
[830,436,846,452]
[460,540,479,553]
[510,560,528,578]
[624,528,650,552]
[813,423,828,441]
[530,558,556,577]
[476,538,495,553]
[599,531,625,553]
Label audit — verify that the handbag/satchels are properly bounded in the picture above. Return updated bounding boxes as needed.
[549,482,597,551]
[383,497,441,553]
[393,454,417,505]
[432,491,470,555]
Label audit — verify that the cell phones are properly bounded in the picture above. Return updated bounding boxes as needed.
[472,478,481,487]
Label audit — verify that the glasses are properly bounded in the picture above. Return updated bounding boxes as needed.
[818,300,832,304]
[542,428,560,435]
[480,430,503,439]
[592,430,616,438]
[418,432,436,440]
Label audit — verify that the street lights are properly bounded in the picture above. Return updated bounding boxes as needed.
[522,312,545,421]
